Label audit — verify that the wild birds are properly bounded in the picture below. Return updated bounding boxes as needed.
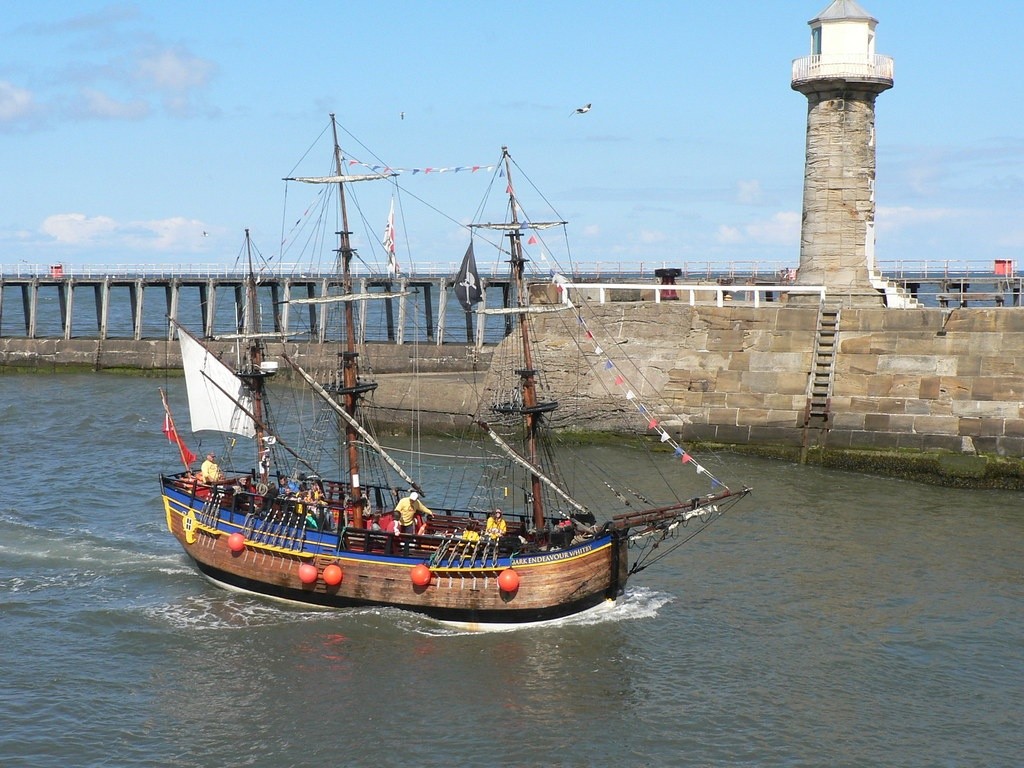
[400,111,405,119]
[569,103,592,117]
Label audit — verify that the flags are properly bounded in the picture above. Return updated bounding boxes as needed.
[453,241,484,311]
[163,415,196,465]
[383,196,401,277]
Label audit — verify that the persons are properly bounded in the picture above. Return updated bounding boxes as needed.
[201,452,506,552]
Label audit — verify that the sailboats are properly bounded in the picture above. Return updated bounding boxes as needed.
[156,110,754,624]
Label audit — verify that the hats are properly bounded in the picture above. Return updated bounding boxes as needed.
[410,491,418,501]
[208,452,215,457]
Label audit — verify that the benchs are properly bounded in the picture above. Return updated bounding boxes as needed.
[220,498,524,560]
[936,292,1004,307]
